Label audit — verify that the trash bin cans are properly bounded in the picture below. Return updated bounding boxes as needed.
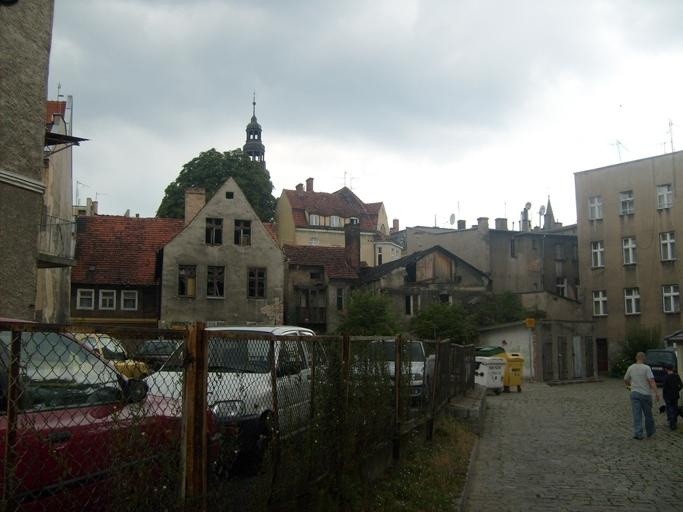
[474,346,525,395]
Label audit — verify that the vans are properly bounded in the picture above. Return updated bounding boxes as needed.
[644,348,677,385]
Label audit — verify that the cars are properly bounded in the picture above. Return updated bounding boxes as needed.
[144,324,328,476]
[0,317,222,510]
[354,338,428,404]
[68,332,151,390]
[132,339,190,370]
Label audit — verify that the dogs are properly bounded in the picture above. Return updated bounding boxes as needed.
[659,405,683,423]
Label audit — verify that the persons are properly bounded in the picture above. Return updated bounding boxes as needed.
[622,352,660,441]
[662,362,683,430]
